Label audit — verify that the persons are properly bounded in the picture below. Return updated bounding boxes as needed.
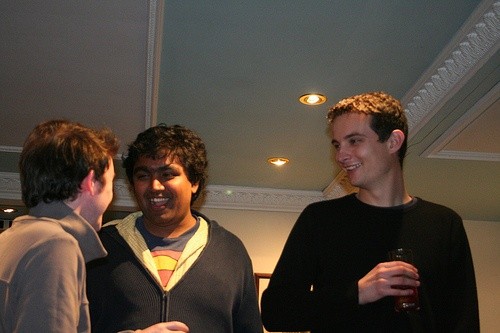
[0,120,190,333]
[86,123,260,333]
[261,92,481,333]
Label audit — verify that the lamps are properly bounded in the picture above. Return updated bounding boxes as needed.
[267,157,290,167]
[298,93,327,106]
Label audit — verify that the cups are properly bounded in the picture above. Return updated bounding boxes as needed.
[389,248,420,314]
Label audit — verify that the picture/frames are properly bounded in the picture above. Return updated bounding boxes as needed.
[255,272,272,307]
